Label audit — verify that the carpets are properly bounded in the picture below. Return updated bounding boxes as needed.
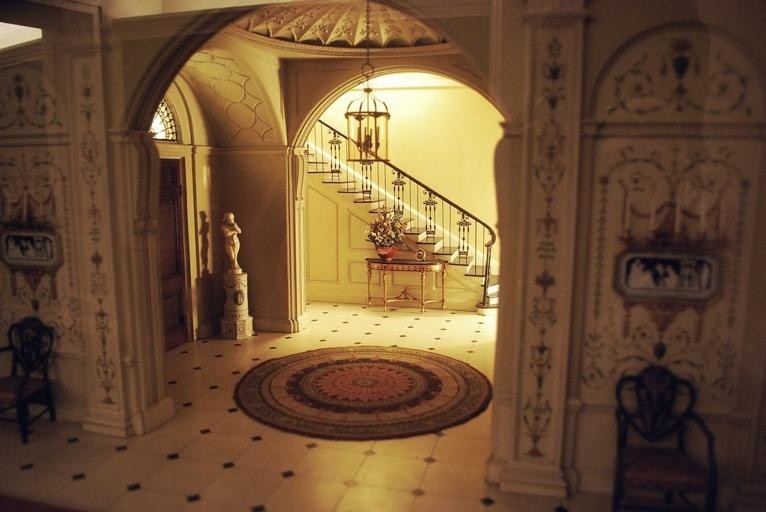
[234,345,492,440]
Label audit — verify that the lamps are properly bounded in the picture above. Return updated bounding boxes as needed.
[345,0,390,163]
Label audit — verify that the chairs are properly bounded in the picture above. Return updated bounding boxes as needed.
[614,366,717,512]
[0,317,56,443]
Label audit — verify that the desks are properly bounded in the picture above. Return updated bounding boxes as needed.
[365,258,448,314]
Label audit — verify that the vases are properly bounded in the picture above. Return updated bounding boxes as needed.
[376,247,397,260]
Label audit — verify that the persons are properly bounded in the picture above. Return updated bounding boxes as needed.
[220,212,243,269]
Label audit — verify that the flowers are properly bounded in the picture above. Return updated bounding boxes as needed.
[365,210,406,247]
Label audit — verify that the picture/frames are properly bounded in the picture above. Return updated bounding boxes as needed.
[615,247,721,303]
[0,227,62,270]
